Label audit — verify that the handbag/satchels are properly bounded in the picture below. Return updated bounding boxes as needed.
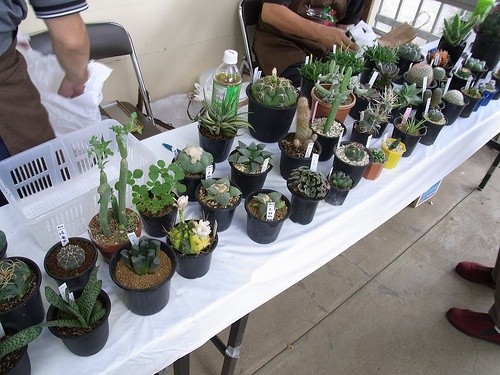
[378,12,430,48]
[15,33,113,174]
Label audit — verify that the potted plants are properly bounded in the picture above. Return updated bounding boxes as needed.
[0,0,500,374]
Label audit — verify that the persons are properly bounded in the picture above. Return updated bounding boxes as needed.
[252,0,364,88]
[0,0,91,192]
[446,246,500,346]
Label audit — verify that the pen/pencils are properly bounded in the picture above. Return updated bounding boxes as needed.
[163,143,180,153]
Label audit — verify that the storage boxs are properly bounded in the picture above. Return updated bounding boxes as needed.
[0,117,161,249]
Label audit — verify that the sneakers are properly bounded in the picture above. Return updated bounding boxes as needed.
[455,262,497,290]
[446,308,500,345]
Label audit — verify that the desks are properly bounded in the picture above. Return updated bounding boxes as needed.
[1,28,499,375]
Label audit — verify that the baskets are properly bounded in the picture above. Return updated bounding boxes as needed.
[0,119,157,252]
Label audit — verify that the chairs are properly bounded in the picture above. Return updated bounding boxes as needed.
[27,21,160,142]
[238,0,265,82]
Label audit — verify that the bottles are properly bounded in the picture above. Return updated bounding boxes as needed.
[211,49,242,122]
